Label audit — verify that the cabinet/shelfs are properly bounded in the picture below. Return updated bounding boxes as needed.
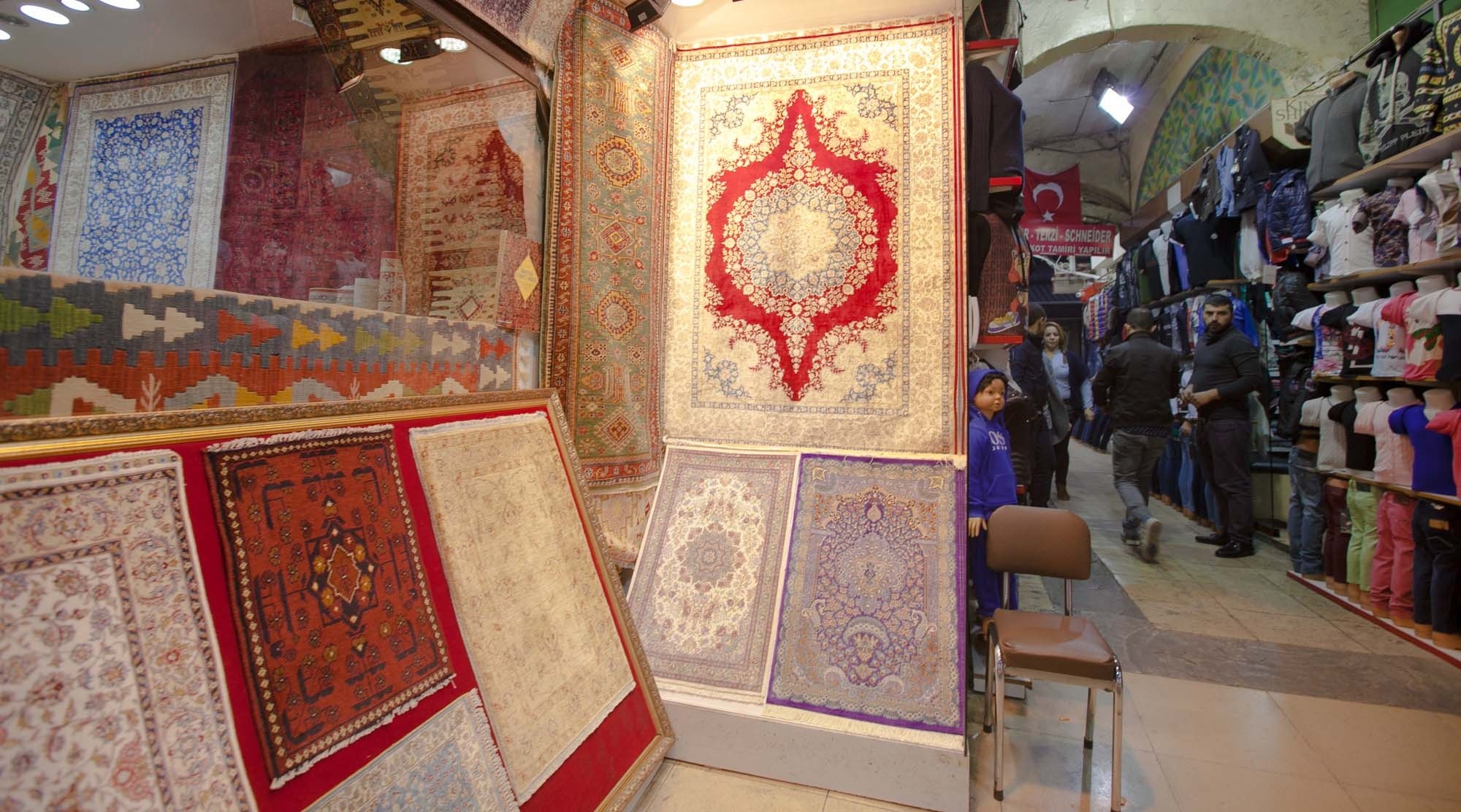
[965,0,1461,703]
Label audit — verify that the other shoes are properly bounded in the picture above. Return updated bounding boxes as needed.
[1122,532,1140,545]
[1057,487,1069,500]
[1142,518,1162,563]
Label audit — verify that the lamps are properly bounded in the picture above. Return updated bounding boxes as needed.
[1091,68,1139,126]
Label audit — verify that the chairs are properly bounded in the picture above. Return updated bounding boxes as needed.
[986,505,1123,812]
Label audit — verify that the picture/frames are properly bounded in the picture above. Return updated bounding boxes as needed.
[1,385,678,812]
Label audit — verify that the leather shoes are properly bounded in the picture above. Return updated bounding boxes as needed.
[1195,533,1228,544]
[1215,541,1255,556]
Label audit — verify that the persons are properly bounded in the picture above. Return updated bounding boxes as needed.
[1148,435,1221,533]
[1070,405,1114,454]
[1130,201,1235,304]
[1181,295,1263,558]
[967,372,1019,640]
[1092,310,1185,562]
[1041,321,1091,500]
[968,49,1035,344]
[1009,304,1058,507]
[1287,19,1461,649]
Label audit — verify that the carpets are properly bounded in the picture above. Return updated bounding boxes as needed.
[545,0,963,734]
[0,0,544,333]
